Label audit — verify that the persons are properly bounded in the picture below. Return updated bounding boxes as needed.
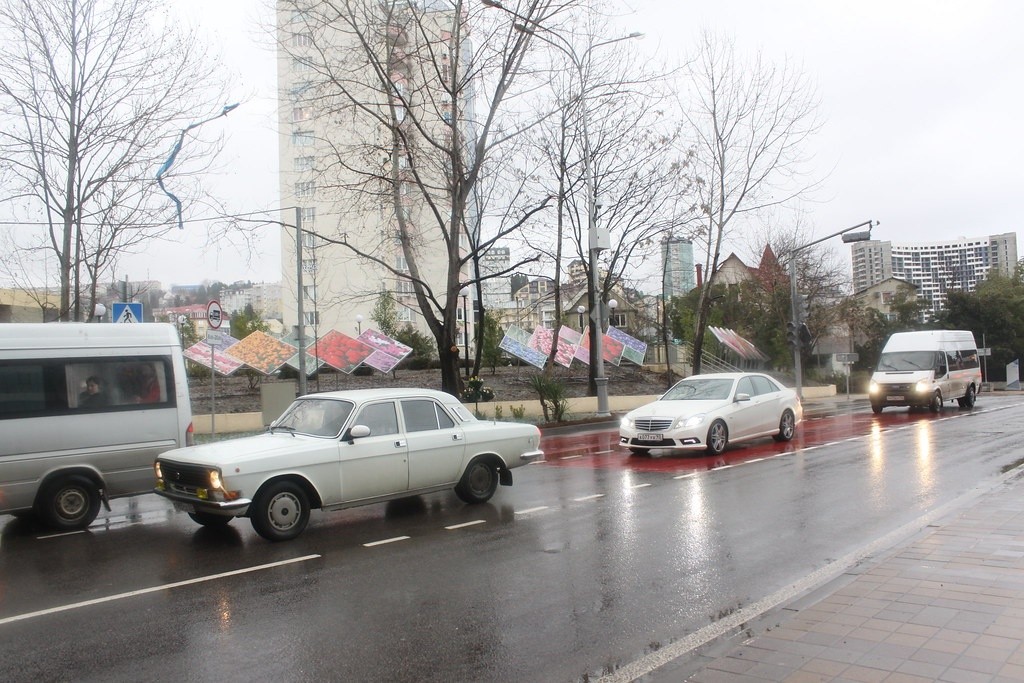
[78,377,105,408]
[138,364,160,403]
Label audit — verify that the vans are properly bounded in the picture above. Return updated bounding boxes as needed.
[867,329,983,413]
[0,322,195,533]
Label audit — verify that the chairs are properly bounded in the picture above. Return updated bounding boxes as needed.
[106,386,121,405]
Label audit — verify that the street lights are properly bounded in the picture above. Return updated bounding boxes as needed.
[608,298,617,329]
[355,315,363,336]
[459,285,472,377]
[93,302,107,323]
[577,305,585,334]
[177,315,187,368]
[481,0,649,419]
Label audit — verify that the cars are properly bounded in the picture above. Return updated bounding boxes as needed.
[617,372,804,456]
[151,388,545,541]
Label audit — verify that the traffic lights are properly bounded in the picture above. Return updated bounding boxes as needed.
[786,321,796,350]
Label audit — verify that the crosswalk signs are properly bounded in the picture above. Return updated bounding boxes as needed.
[113,301,144,322]
